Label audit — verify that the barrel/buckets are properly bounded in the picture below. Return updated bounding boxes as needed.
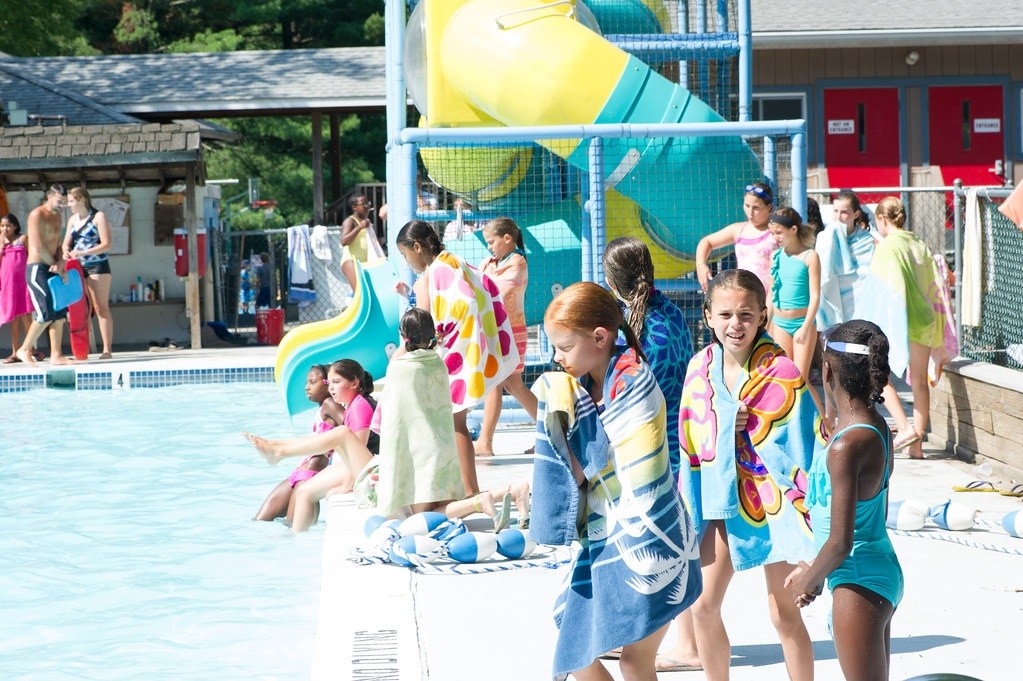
[256,308,285,345]
[173,227,208,277]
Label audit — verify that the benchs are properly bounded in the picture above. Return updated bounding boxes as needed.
[87,297,205,354]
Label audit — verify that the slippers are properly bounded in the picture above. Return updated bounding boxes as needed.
[492,493,511,533]
[998,483,1023,497]
[655,654,703,672]
[517,514,530,529]
[953,481,1002,493]
[892,435,919,451]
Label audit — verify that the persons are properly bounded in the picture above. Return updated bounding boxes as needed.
[535,182,961,681]
[339,193,388,294]
[378,168,438,229]
[444,198,486,241]
[396,214,538,504]
[0,184,113,368]
[372,306,531,535]
[243,358,379,534]
[240,259,253,314]
[254,252,279,311]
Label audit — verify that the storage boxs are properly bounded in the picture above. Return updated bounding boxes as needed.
[172,228,205,274]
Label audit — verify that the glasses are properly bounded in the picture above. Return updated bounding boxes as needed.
[746,185,770,200]
[357,201,372,207]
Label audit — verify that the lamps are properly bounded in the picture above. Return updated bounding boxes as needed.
[906,53,919,65]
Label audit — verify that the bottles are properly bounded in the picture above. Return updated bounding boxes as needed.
[145,274,154,288]
[130,284,137,302]
[144,287,151,302]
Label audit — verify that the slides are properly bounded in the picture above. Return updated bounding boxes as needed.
[271,0,777,434]
